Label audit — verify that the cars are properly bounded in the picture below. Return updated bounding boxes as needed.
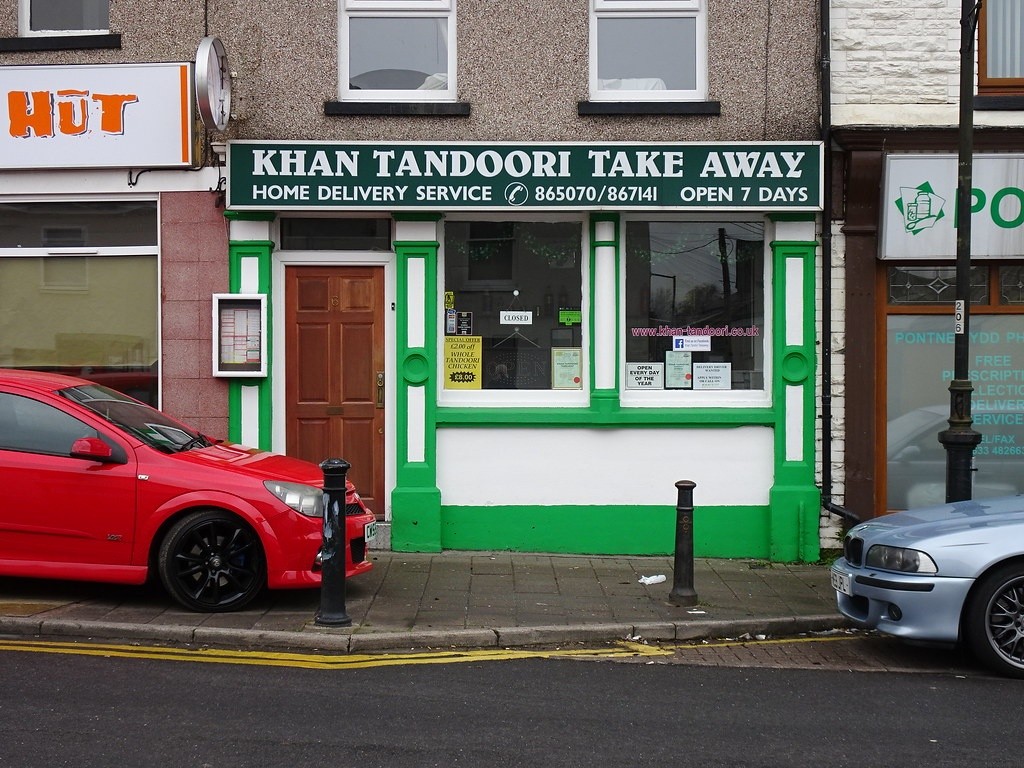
[831,493,1024,680]
[0,369,376,614]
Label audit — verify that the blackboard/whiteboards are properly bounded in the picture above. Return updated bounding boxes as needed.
[482,347,551,390]
